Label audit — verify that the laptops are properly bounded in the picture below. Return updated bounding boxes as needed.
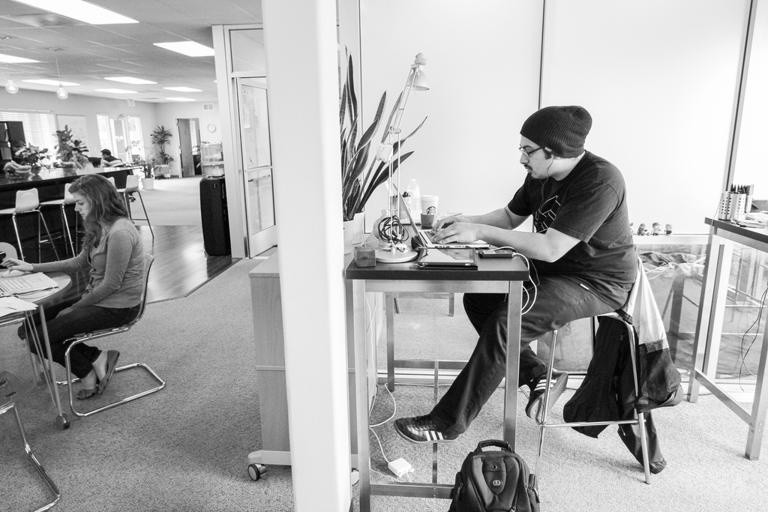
[393,184,489,249]
[0,272,58,297]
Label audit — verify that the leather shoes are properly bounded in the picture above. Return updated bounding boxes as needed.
[75,349,120,400]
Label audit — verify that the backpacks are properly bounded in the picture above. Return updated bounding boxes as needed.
[448,439,543,512]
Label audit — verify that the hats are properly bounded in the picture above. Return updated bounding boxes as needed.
[520,106,594,159]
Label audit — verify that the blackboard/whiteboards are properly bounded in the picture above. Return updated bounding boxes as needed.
[200,174,232,256]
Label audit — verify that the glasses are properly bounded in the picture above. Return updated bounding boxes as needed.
[518,146,546,157]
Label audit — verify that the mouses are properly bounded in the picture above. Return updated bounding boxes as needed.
[2,269,24,278]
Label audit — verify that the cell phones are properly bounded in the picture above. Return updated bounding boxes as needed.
[479,248,512,258]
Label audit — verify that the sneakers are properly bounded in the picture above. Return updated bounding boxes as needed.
[525,368,569,420]
[393,414,460,444]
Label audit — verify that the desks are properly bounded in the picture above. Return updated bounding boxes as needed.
[345,219,531,512]
[1,266,73,430]
[685,213,768,464]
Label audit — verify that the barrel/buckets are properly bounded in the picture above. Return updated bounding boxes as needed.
[199,142,224,180]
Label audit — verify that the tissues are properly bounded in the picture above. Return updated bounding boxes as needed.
[354,234,376,268]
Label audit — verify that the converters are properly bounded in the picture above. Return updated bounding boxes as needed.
[389,458,411,478]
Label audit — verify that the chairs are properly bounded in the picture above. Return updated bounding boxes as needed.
[1,369,62,511]
[533,254,658,488]
[54,252,165,417]
[107,175,156,239]
[0,242,18,268]
[1,188,60,262]
[39,183,86,263]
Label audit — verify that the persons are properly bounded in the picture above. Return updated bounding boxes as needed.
[393,104,640,444]
[100,148,127,216]
[0,173,147,401]
[53,148,90,169]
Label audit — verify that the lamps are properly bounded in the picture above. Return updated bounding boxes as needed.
[49,46,71,101]
[0,35,19,96]
[374,51,430,264]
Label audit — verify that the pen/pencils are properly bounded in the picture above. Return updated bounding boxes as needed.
[730,184,751,194]
[393,191,409,198]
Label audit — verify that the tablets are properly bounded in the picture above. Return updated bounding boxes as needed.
[420,247,476,266]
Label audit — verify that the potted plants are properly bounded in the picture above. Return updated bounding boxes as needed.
[338,44,388,257]
[353,92,427,246]
[149,126,175,179]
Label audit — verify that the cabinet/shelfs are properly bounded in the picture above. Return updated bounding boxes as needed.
[246,249,291,454]
